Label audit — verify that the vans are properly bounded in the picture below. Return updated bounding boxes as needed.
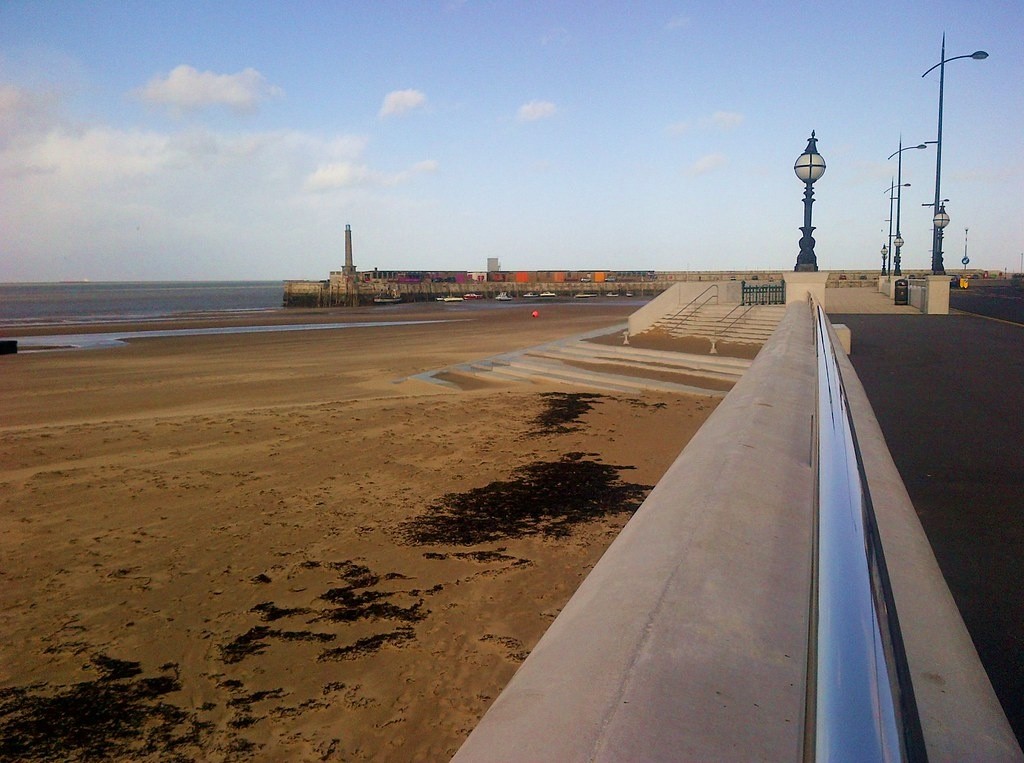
[605,276,617,282]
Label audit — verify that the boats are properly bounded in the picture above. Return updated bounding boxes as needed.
[373,289,403,305]
[522,291,539,298]
[574,291,598,298]
[463,293,483,300]
[540,290,555,297]
[495,291,513,301]
[434,296,464,302]
[626,293,634,297]
[606,292,619,297]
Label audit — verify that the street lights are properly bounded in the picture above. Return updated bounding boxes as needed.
[793,129,827,272]
[880,244,888,276]
[932,201,950,275]
[920,28,990,274]
[894,231,905,276]
[887,176,911,279]
[883,132,928,277]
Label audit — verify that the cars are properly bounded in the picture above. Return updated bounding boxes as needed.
[839,274,846,280]
[752,276,758,280]
[432,277,456,283]
[965,273,979,279]
[731,276,736,280]
[580,277,591,282]
[909,274,915,279]
[947,274,961,288]
[860,276,867,280]
[920,273,929,279]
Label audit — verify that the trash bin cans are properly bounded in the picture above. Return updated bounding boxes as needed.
[894,279,908,305]
